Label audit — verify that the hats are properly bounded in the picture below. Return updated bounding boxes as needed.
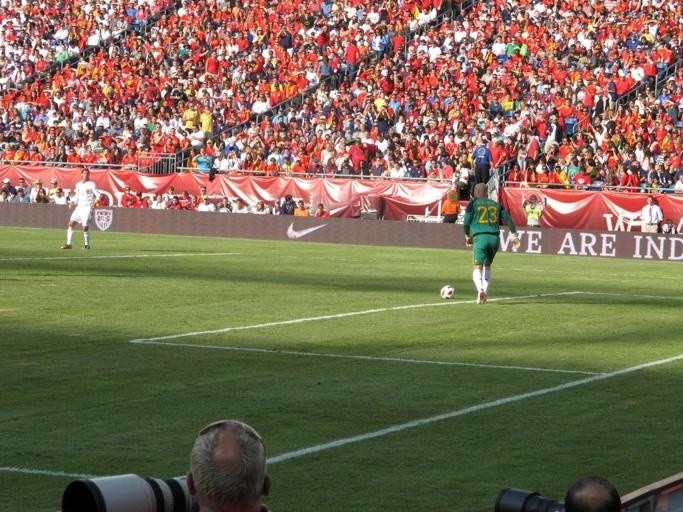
[135,192,142,196]
[285,194,292,200]
[1,177,62,195]
[124,186,131,192]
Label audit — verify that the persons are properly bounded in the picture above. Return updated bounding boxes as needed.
[185,418,269,510]
[462,182,517,303]
[1,0,683,235]
[60,168,101,249]
[563,475,624,510]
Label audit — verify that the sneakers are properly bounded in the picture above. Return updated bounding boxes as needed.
[476,287,488,305]
[61,243,74,249]
[82,242,91,251]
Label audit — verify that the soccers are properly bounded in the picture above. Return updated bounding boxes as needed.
[441,285,455,300]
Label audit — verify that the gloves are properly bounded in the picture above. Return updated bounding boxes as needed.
[513,235,523,250]
[465,235,476,248]
